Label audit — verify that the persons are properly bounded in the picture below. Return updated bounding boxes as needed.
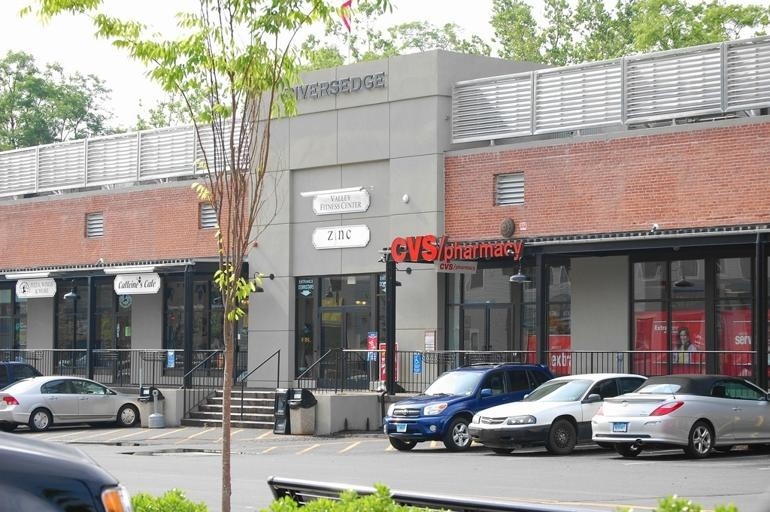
[672,324,698,364]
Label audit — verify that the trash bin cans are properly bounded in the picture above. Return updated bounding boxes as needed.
[287,389,318,435]
[137,386,165,427]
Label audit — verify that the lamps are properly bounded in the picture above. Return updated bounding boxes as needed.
[504,259,537,286]
[57,280,84,302]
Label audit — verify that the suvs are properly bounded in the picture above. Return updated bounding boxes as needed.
[382,362,556,452]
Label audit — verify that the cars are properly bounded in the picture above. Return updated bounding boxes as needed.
[0,361,142,512]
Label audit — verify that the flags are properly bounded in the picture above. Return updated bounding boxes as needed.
[340,0,353,31]
